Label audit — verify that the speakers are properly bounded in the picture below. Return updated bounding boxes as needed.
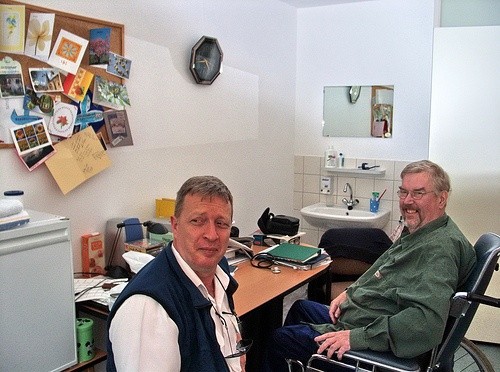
[105,217,144,271]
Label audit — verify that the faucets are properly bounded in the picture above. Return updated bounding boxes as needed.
[342,182,359,209]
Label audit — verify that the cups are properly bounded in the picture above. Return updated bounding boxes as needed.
[370,196,380,212]
[326,188,334,207]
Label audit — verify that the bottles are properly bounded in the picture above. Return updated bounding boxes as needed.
[338,153,344,168]
[3,190,24,209]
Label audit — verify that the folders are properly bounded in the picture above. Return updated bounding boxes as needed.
[267,243,321,263]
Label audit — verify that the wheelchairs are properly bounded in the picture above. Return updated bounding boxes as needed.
[284,232,500,372]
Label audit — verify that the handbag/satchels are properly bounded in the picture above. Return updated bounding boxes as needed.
[257,207,300,237]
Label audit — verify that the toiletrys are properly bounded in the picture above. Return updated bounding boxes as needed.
[371,191,379,201]
[325,144,337,169]
[338,152,344,168]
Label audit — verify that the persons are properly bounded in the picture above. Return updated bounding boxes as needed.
[259,157,476,372]
[106,175,250,372]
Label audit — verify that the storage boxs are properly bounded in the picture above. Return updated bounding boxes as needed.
[79,233,104,276]
[125,239,167,258]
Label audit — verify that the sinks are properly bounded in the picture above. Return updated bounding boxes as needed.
[300,203,390,231]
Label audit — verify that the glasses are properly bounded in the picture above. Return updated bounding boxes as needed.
[396,190,437,200]
[216,311,253,358]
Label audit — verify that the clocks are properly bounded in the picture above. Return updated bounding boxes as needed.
[321,83,396,137]
[190,35,224,86]
[348,86,364,104]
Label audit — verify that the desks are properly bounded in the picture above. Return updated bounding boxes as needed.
[76,238,333,372]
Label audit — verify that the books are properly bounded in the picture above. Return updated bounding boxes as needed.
[223,245,238,260]
[258,241,322,265]
